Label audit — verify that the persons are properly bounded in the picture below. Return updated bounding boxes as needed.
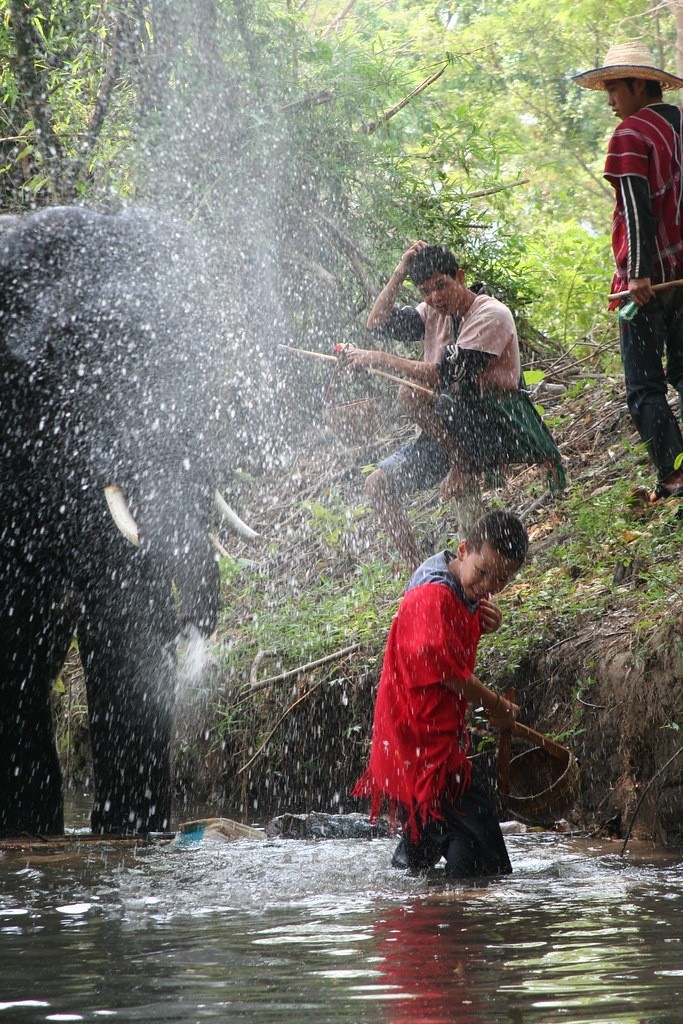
[567,44,683,499]
[353,511,530,876]
[330,237,522,584]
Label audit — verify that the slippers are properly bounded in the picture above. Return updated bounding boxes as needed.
[649,481,683,501]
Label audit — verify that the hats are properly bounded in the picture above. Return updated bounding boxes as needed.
[571,42,683,91]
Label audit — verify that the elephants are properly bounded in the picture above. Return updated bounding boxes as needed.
[0,203,265,838]
